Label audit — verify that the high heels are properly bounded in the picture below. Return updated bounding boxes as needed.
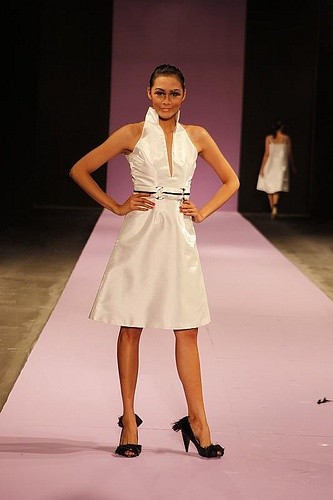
[171,416,225,458]
[115,414,143,457]
[271,204,282,220]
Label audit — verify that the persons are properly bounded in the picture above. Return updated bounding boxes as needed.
[69,64,240,458]
[257,119,297,217]
